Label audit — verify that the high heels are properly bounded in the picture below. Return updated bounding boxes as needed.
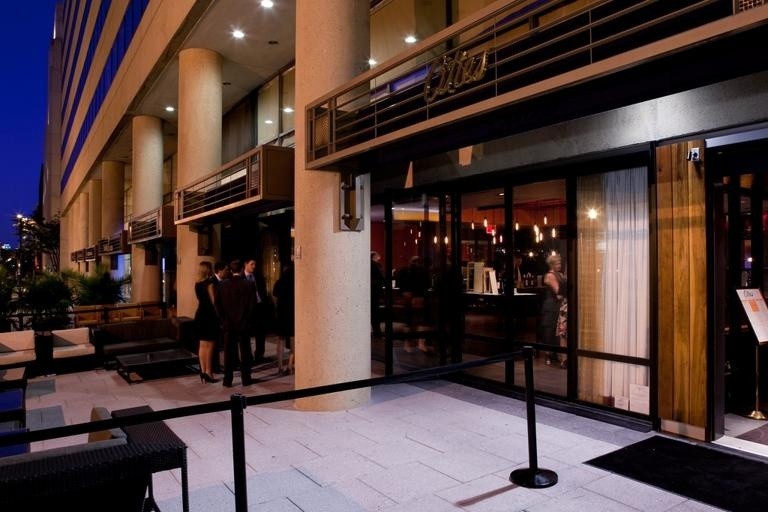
[200,373,219,385]
[403,346,436,354]
[278,366,295,376]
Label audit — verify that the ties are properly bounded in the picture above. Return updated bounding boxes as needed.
[249,276,252,281]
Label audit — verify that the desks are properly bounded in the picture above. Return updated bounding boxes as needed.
[379,287,541,359]
[116,346,199,384]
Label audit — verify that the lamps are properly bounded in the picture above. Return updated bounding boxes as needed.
[469,205,557,257]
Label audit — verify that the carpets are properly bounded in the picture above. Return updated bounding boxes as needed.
[582,434,768,512]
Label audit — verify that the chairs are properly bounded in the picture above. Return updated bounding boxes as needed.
[1,367,31,453]
[3,401,191,511]
[51,326,96,359]
[1,329,37,365]
[90,317,194,372]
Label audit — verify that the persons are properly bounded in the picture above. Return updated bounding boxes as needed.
[370,252,466,366]
[498,254,525,293]
[195,253,295,388]
[539,249,570,366]
[553,273,572,368]
